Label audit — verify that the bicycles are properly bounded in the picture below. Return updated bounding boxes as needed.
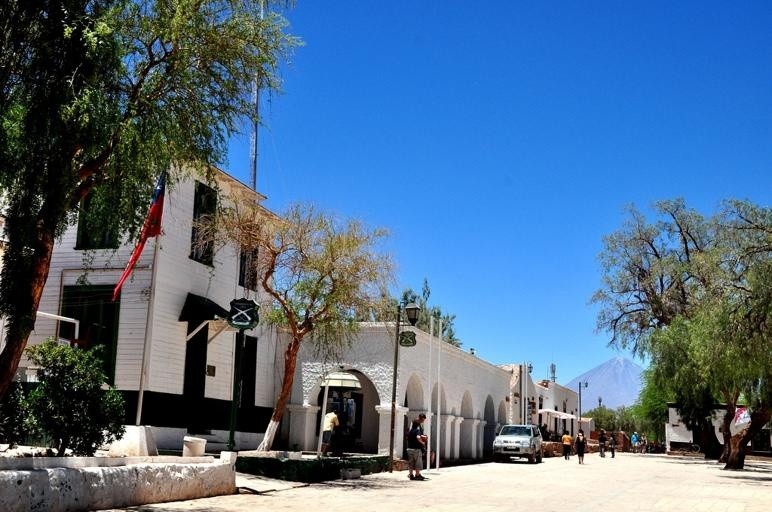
[679,444,700,456]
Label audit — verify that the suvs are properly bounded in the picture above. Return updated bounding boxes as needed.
[493,424,543,464]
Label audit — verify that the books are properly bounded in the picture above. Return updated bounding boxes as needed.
[421,435,427,443]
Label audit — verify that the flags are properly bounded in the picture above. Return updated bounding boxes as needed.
[110,158,169,305]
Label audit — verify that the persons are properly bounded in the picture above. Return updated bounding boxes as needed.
[407,413,427,478]
[575,428,587,465]
[540,422,548,440]
[608,432,618,458]
[320,405,339,457]
[630,431,666,455]
[598,432,608,458]
[406,423,429,481]
[561,430,574,461]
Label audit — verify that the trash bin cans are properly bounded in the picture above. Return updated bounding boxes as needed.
[182,436,208,457]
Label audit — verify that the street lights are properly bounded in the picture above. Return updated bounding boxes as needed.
[578,381,588,428]
[389,301,421,471]
